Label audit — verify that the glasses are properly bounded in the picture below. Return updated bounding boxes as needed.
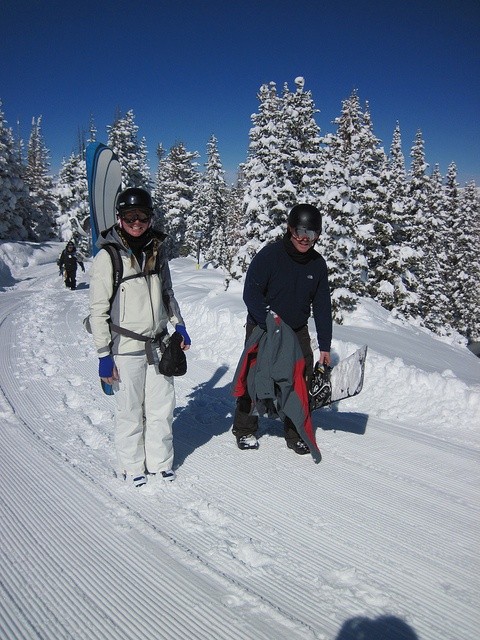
[68,245,73,248]
[292,227,318,241]
[115,206,152,221]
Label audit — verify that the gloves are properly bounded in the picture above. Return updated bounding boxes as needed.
[82,266,85,272]
[99,356,115,376]
[176,326,191,345]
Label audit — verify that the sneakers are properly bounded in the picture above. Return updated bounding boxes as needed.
[288,439,309,455]
[133,474,147,488]
[72,287,77,290]
[232,430,258,449]
[66,284,70,287]
[162,471,175,482]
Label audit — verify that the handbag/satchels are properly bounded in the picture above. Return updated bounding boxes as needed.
[159,331,187,377]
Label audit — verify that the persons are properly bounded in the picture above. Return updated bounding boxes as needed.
[56,232,87,291]
[88,186,192,485]
[232,204,333,454]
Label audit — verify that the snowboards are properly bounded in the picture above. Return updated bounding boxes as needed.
[59,253,68,282]
[86,142,121,257]
[310,343,368,411]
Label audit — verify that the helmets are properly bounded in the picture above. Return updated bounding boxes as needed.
[116,188,152,215]
[288,204,322,230]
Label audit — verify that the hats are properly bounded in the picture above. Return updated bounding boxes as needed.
[68,242,74,245]
